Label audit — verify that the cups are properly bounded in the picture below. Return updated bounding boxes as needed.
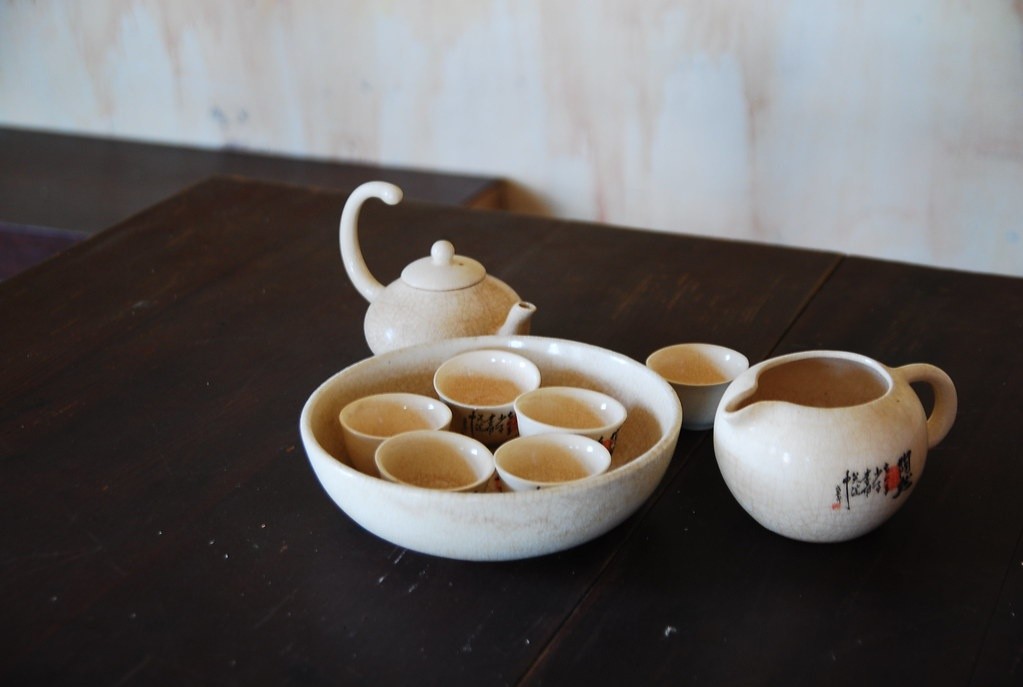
[375,430,495,492]
[493,433,611,492]
[433,350,541,448]
[339,393,453,479]
[646,343,749,430]
[514,386,627,457]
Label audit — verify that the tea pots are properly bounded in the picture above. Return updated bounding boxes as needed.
[339,180,536,355]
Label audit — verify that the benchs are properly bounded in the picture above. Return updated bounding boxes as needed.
[0,131,507,281]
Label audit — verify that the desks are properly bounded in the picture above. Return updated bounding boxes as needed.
[0,175,1023,687]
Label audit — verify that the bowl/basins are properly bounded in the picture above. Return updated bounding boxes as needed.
[300,335,683,562]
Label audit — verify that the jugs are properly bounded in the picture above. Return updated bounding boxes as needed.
[714,350,957,542]
